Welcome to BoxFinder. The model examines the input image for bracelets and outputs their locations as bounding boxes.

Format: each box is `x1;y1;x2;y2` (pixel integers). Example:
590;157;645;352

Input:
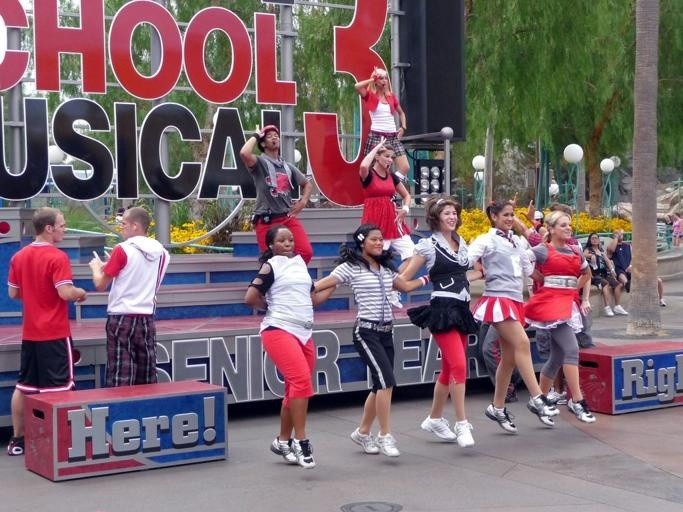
251;133;261;140
372;74;378;82
400;126;407;131
418;275;430;288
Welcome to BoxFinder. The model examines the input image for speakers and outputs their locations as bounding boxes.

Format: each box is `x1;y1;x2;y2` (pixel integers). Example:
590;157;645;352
397;0;467;144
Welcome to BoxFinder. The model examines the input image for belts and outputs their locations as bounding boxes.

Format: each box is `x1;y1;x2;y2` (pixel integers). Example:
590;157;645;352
263;310;313;331
369;130;398;138
353;318;394;333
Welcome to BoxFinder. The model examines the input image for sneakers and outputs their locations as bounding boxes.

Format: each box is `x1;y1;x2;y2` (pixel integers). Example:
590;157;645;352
614;304;628;316
605;306;614;319
392;289;403;310
485;403;520;432
453;420;475;449
530;395;561;417
350;427;379;455
568;398;596;423
525;399;555;428
288;437;312;466
658;298;666;307
374;431;399;458
270;438;298;466
548;387;567;406
420;416;458;441
503;390;518;404
6;436;25;458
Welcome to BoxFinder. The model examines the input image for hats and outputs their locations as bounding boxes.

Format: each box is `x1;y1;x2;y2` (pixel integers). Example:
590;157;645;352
260;124;279;133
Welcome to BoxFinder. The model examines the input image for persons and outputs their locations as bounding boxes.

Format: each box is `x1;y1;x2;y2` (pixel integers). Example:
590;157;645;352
665;215;673;226
509;192;597;405
672;213;683;247
524;210;597;424
534;211;545;225
240;123;313;267
88;208;171;388
358;136;415;274
7;207;87;456
478;322;521;403
468;200;561;433
399;197;483;448
315;225;431;457
244;225;344;469
607;228;667;306
355;66;410;183
583;233;630;317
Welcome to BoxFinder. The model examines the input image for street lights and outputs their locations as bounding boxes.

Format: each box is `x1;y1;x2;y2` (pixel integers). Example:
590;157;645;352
600;156;620;233
472;155;485;209
564;144;583;235
